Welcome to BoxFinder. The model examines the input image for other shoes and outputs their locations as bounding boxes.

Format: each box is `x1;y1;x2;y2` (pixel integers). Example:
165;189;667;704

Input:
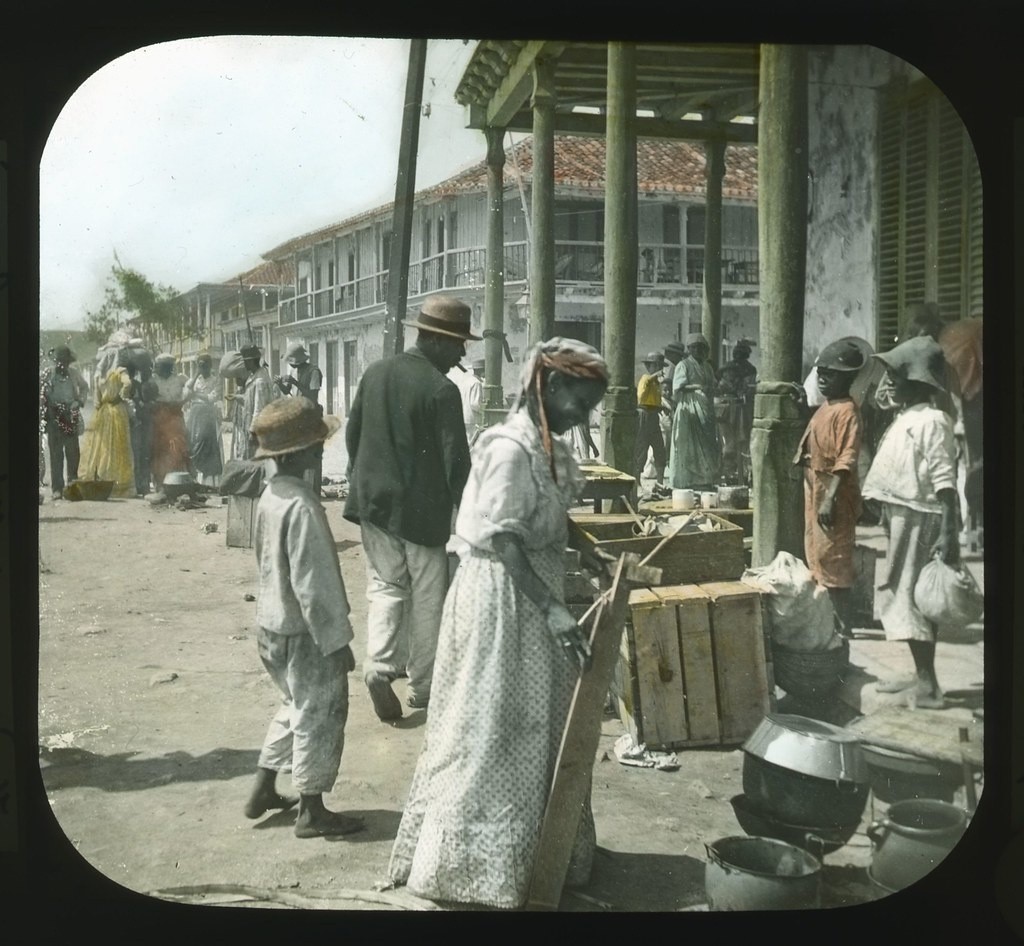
637;485;652;500
652;486;673;499
52;491;62;499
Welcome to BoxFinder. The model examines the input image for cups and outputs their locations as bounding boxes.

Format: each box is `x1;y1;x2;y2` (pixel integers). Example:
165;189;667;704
672;489;696;512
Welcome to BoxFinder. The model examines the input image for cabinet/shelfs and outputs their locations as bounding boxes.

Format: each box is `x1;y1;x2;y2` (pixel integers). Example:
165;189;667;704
605;579;771;750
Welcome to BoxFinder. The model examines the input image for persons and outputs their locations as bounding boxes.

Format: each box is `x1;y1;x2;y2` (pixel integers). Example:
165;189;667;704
632;334;760;492
42;332;322;499
244;395;367;839
342;296;482;721
860;336;984;708
391;337;618;909
790;337;866;637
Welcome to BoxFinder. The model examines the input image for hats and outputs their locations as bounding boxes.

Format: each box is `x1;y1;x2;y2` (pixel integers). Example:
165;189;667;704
240;344;261;361
662;340;687;357
642;352;669;367
154;353;175;365
400;296;483;341
869;336;947;395
252;397;341;460
687;333;706;347
810;342;863;370
48;346;76;363
281;343;309;365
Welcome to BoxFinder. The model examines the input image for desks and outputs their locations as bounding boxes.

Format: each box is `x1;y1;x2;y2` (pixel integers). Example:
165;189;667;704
576;458;639;514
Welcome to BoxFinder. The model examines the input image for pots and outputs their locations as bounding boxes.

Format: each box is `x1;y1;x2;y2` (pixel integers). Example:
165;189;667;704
863;742;982;806
704;836;822;911
69;480;115;501
740;750;870;831
162;482;199;504
731;793;863;859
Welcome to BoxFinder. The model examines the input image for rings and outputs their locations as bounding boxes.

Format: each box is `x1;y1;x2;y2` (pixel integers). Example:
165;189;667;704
564;641;570;647
